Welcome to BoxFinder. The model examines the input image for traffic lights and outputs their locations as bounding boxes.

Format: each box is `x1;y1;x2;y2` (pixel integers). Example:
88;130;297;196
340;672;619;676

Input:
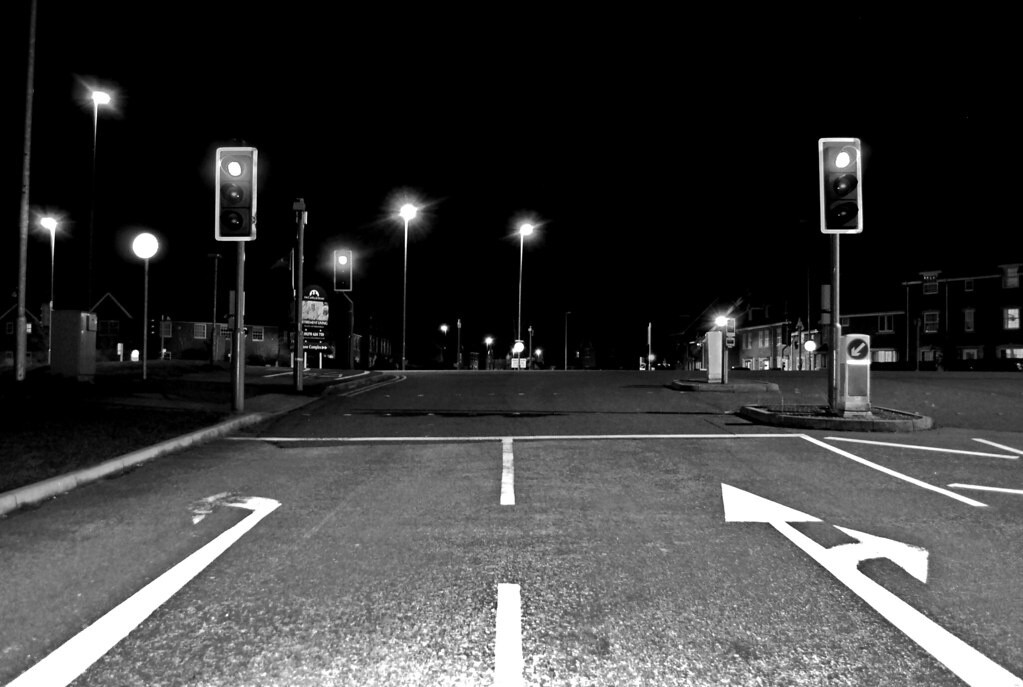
215;147;257;241
334;249;353;292
819;138;863;234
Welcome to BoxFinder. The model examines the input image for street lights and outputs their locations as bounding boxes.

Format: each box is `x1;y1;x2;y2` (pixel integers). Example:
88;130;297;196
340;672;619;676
401;204;416;370
84;90;111;313
564;311;572;370
518;224;534;371
40;217;57;366
133;232;160;378
